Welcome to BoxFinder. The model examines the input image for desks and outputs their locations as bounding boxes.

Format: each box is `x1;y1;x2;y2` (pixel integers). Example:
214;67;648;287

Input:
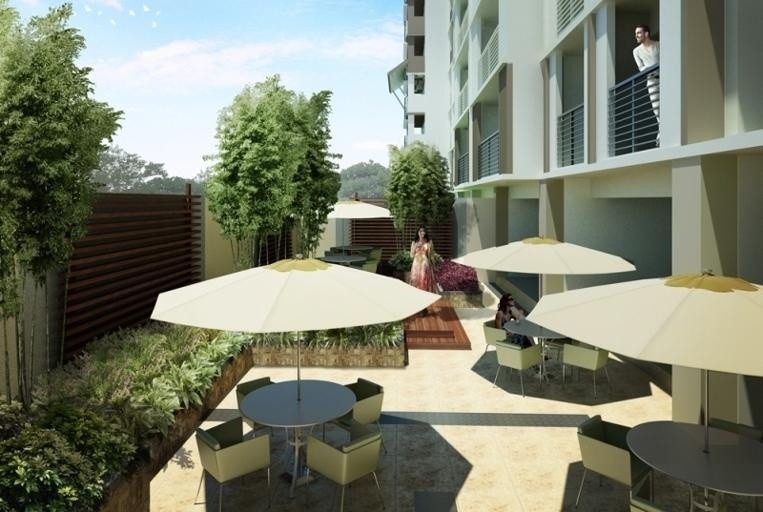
626;420;763;512
239;380;356;500
503;319;568;384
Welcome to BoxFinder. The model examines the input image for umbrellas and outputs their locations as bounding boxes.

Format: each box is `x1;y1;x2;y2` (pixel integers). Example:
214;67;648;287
148;257;442;400
524;267;763;506
450;235;635;303
316;199;396;246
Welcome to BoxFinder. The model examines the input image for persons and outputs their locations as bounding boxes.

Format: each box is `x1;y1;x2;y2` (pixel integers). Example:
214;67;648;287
631;24;660;149
495;293;541;373
409;225;435;292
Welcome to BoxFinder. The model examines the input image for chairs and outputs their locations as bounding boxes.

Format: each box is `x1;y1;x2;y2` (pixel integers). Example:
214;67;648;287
562;343;614;400
630;473;667;512
194;416;271;512
575;415;654;509
306;419;386;511
235;376;277;438
322;378;388;455
315;247;374;269
482;319;518;353
492;340;543;397
